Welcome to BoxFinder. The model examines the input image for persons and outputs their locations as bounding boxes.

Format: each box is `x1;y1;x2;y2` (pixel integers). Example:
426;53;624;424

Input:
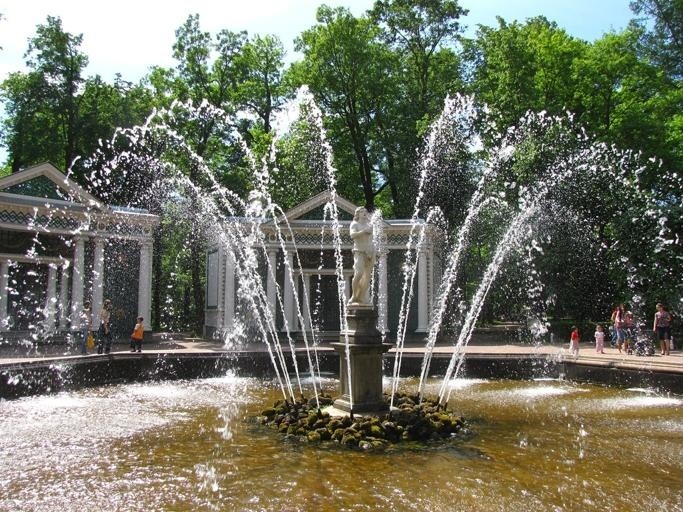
79;301;92;355
653;303;672;355
610;304;634;355
348;206;375;304
130;317;144;352
98;299;113;354
568;326;579;356
595;325;605;354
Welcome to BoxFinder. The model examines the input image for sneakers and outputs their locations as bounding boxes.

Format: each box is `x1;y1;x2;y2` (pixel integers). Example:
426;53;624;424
132;349;141;352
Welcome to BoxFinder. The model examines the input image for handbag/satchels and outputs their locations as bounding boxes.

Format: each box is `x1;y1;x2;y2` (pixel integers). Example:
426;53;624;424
131;329;141;340
88;336;94;348
